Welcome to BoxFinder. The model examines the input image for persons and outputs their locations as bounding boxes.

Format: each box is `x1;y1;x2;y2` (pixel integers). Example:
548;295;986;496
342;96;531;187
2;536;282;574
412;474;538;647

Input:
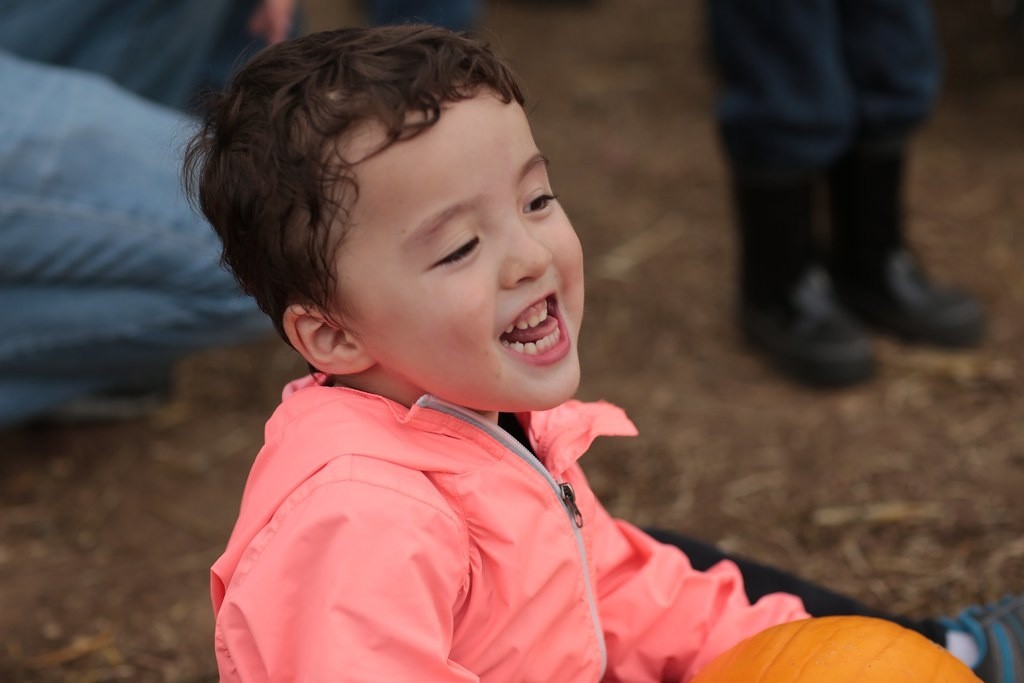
180;23;1024;683
0;0;296;433
701;0;993;387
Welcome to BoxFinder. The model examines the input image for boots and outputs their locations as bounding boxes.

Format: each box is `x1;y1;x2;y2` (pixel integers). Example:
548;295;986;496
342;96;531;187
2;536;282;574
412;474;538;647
733;158;878;382
830;145;986;342
